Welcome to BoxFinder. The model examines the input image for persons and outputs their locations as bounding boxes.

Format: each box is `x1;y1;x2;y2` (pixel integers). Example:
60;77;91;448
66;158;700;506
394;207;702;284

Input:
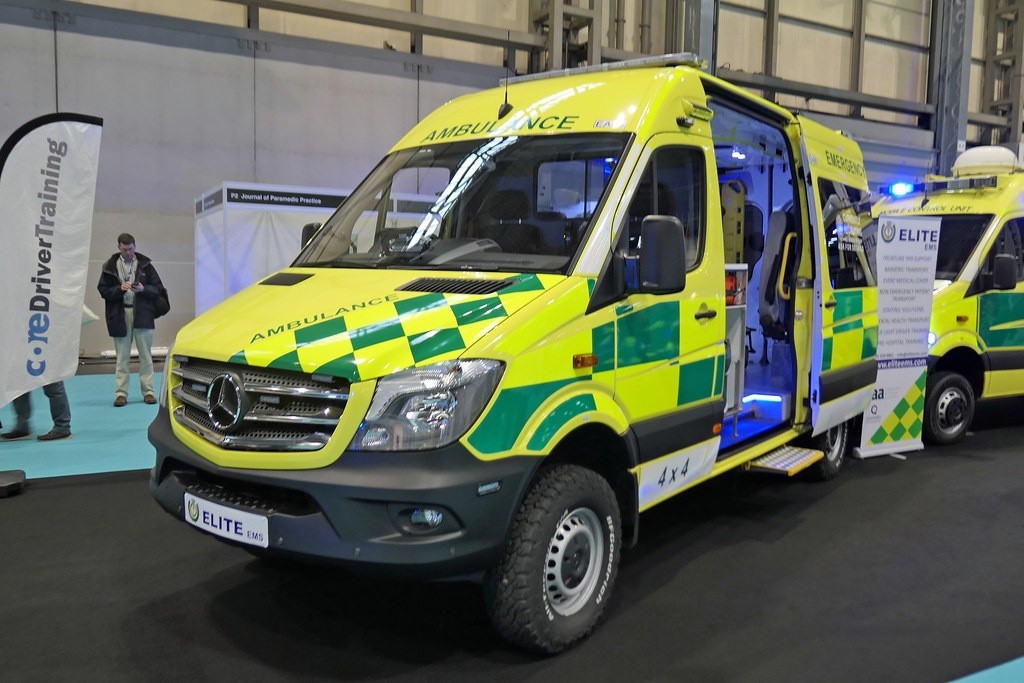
0;381;73;442
97;231;159;406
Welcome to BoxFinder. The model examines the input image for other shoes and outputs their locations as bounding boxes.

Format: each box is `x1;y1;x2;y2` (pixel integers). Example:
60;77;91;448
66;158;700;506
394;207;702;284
36;430;71;439
0;433;32;441
144;395;156;404
114;397;126;406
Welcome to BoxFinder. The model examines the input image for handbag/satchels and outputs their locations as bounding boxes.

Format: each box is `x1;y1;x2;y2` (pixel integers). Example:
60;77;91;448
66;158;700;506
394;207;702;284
153;288;170;317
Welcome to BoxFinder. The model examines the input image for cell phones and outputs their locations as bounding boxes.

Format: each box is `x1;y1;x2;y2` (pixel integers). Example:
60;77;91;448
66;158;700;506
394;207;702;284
131;281;140;289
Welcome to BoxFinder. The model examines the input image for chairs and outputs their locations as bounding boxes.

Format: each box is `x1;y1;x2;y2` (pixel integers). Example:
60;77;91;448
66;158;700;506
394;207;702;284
624;183;676;248
478;189;545;255
960;236;998;272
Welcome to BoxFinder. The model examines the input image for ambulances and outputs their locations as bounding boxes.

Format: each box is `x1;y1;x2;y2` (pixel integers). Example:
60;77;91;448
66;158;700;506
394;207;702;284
862;145;1023;448
146;49;879;659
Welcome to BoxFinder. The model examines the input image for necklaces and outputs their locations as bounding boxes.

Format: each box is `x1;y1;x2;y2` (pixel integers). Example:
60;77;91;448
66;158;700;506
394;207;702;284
119;258;135;284
